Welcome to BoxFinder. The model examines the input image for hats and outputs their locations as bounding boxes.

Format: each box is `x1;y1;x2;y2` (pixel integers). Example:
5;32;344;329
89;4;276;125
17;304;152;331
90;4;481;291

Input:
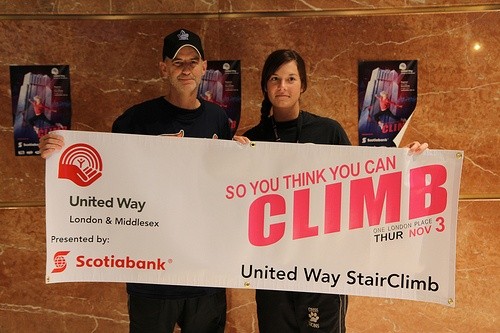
164;29;204;61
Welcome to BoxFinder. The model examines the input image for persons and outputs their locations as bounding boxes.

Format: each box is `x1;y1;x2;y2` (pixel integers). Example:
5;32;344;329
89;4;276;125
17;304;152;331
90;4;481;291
28;96;63;135
374;90;407;131
38;28;236;333
200;91;236;129
233;49;429;333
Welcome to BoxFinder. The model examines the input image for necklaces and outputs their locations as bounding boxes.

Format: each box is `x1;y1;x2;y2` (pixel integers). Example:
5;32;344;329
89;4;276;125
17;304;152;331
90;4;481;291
271;114;303;144
